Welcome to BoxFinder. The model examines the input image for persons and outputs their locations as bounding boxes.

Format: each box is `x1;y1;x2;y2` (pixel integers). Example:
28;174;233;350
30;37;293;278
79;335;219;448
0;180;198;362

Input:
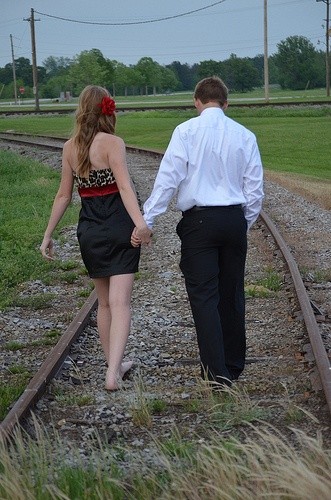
131;77;264;388
39;84;152;392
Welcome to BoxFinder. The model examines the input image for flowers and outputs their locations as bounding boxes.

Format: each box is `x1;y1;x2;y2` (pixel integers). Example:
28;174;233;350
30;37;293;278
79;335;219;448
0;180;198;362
97;96;116;116
